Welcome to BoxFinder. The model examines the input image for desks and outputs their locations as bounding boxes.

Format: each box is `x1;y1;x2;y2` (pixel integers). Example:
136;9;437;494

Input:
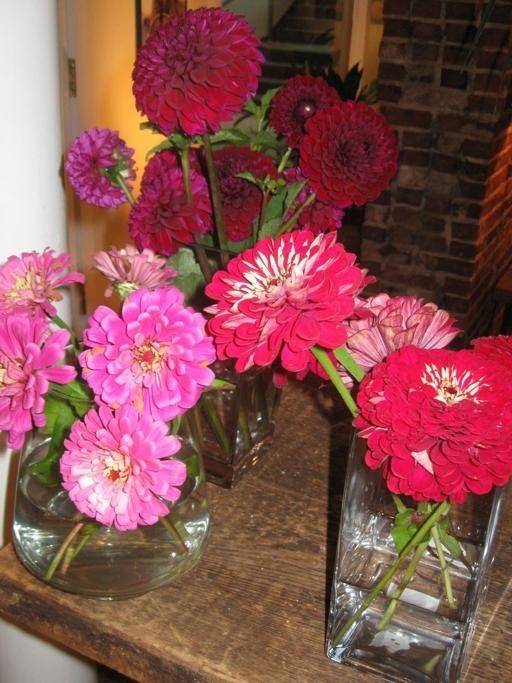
0;302;512;683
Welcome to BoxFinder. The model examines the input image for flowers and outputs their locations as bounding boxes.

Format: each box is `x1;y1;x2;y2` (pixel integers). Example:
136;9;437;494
60;6;405;463
2;247;218;583
199;226;512;650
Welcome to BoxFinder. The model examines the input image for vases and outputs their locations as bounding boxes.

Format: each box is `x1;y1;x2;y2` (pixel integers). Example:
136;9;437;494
11;389;208;602
320;431;504;682
165;341;284;485
